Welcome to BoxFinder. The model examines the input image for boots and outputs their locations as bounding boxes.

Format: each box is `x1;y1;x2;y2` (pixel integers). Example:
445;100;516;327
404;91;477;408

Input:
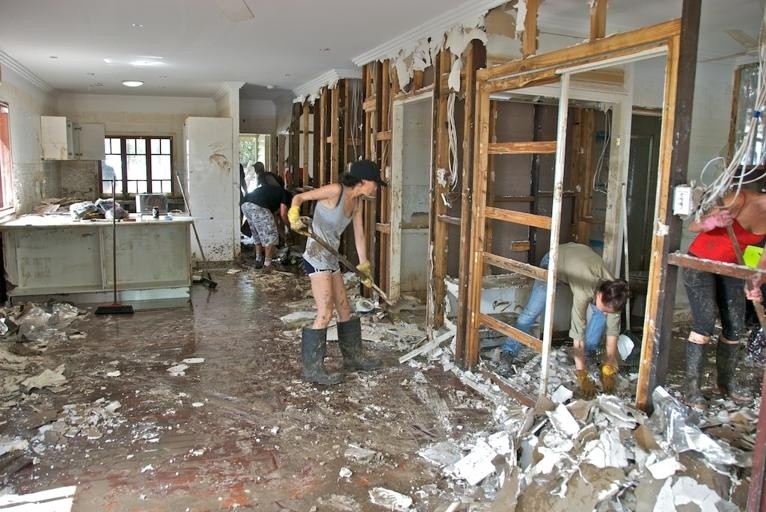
716;332;754;403
299;325;345;386
336;317;383;372
685;331;712;409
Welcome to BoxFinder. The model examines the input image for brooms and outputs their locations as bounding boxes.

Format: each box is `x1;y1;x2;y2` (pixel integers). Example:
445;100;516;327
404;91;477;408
94;176;135;314
176;174;218;288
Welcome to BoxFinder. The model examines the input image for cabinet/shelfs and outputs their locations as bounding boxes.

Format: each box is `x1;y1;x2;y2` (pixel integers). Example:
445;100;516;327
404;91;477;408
65;116;105;162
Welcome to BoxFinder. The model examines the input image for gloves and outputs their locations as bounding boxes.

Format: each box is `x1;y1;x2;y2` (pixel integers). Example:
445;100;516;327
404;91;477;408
602;363;619;394
287;206;306;231
700;209;734;233
355;261;375;288
574;369;596;399
743;280;765;303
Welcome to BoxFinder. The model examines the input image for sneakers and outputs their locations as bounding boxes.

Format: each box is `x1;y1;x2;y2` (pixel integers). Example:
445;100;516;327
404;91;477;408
255;259;264;268
499;349;515;377
262;263;279;274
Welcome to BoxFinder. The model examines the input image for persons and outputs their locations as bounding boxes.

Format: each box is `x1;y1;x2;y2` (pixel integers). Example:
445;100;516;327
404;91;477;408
679;188;765;412
494;240;634;402
285;158;389;387
251;161;286;218
239;184;303;270
238;163;247;224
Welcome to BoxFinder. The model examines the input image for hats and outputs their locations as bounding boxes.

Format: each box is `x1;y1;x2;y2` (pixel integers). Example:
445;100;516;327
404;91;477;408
349;160;388;188
